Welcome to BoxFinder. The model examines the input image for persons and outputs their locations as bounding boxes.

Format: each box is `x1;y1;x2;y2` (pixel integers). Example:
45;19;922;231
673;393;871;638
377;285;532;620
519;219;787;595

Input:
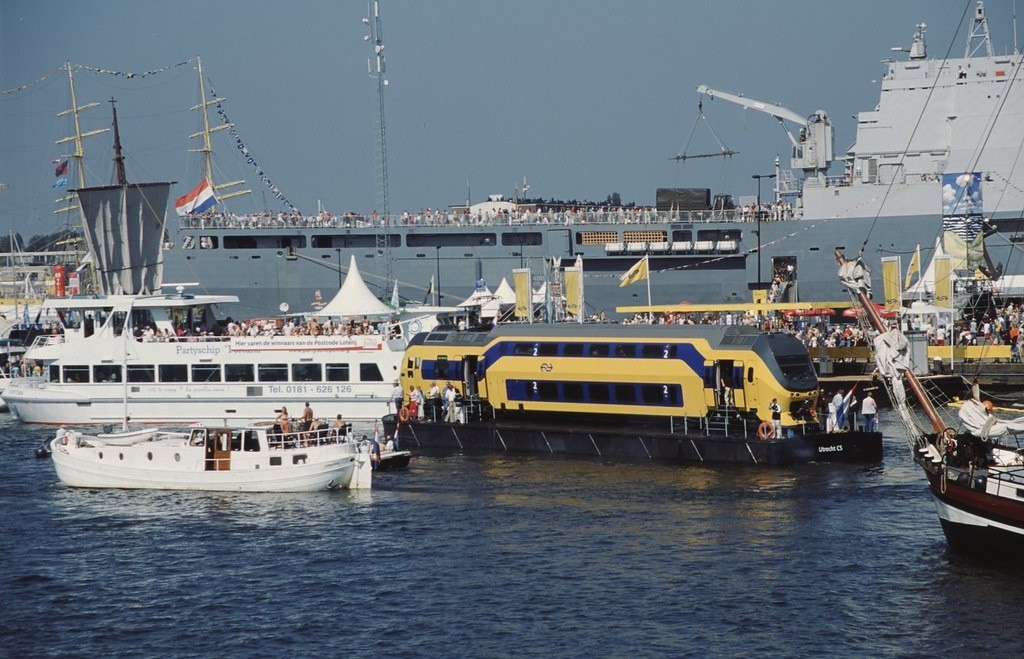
861;392;877;432
896;302;1024;363
737;200;792;223
457;319;466;332
6;356;45;378
134;316;401;343
507;311;881;350
358;435;397;456
384;378;465;425
769;399;784;439
816;389;845;432
175;203;660;232
275;402;345;446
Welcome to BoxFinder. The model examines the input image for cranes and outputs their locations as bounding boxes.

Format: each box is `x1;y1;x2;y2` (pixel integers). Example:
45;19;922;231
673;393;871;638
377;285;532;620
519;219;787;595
669;84;835;179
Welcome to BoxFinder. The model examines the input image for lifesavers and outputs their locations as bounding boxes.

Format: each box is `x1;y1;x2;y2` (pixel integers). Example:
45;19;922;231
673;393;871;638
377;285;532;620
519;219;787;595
758;422;774;438
399;407;410;422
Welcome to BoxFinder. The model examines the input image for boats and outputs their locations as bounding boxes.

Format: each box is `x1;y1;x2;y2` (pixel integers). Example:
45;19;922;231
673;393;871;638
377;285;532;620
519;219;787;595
0;0;1024;424
833;249;1024;567
373;449;411;474
381;322;883;466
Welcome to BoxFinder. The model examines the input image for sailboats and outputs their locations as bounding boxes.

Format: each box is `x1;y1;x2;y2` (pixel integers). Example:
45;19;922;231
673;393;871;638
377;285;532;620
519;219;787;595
50;328;373;491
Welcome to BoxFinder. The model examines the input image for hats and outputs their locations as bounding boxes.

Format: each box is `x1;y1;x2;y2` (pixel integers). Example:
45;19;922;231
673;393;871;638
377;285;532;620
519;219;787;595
1010;302;1014;306
971;318;978;321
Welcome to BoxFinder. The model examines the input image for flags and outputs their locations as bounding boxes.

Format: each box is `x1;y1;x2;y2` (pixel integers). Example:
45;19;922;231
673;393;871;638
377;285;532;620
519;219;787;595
373;421;382;472
934;255;953;308
836;382;859;430
564;266;584;313
175;177;221;225
51;157;68;188
880;256;903;311
618;253;649;288
905;243;921;290
393;421;400;452
512;269;532;317
24;303;30;329
